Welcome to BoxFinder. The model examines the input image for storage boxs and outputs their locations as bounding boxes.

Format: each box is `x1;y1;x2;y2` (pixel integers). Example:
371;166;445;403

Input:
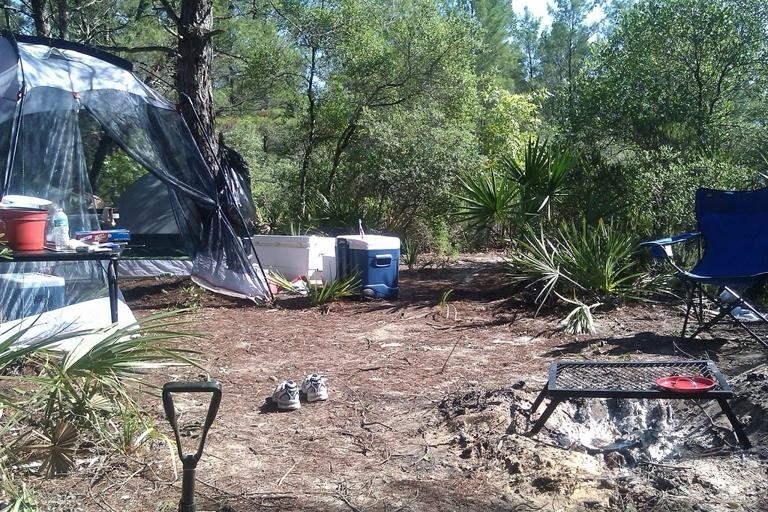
335;234;401;301
1;273;66;321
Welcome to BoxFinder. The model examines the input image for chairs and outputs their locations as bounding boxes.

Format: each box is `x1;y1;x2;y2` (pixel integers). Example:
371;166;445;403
640;188;768;349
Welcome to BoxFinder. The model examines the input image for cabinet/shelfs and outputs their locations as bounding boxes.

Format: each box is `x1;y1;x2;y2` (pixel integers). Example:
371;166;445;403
251;235;336;286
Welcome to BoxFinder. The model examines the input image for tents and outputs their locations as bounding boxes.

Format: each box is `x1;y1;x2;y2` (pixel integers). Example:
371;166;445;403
0;25;271;323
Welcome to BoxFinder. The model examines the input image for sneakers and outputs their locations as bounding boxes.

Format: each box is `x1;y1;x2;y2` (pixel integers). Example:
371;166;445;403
271;373;329;411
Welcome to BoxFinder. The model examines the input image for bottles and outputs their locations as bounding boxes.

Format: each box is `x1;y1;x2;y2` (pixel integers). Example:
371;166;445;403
52;208;69;251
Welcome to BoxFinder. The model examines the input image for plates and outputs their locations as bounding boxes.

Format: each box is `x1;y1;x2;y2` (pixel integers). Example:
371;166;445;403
656;376;717;394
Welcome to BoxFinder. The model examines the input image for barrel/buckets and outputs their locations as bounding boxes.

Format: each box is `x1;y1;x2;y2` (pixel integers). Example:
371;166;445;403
1;209;49;251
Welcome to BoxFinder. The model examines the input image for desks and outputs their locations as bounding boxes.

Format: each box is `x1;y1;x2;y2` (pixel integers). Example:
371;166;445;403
1;252;120;323
530;359;752;450
